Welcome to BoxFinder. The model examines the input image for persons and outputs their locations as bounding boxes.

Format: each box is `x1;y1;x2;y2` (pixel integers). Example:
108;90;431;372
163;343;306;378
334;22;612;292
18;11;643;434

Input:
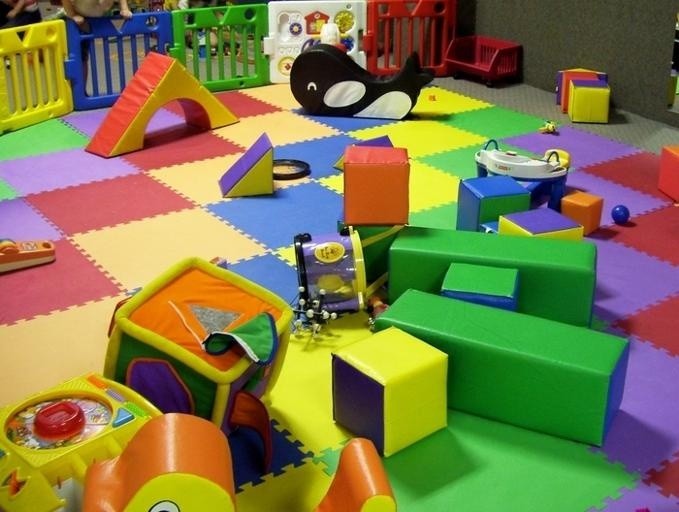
0;0;239;97
0;0;240;96
